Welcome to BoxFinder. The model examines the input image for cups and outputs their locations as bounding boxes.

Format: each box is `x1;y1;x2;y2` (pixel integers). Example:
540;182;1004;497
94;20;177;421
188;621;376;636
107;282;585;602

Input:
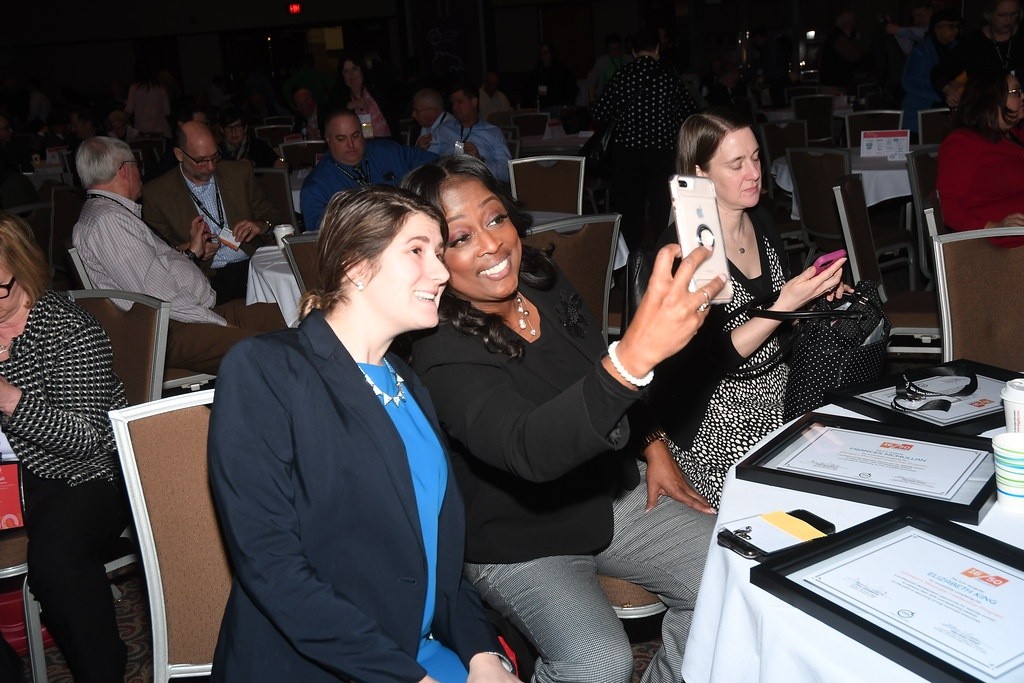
1000;378;1024;432
273;224;295;251
991;432;1024;509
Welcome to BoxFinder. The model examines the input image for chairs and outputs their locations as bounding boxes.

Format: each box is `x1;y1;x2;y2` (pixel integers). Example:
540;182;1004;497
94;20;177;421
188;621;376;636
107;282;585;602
0;68;1024;683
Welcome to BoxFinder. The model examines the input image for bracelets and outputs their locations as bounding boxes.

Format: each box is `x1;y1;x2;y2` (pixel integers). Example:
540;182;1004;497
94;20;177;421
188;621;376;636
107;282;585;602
264;218;271;226
639;429;670;454
608;341;654;386
184;248;199;264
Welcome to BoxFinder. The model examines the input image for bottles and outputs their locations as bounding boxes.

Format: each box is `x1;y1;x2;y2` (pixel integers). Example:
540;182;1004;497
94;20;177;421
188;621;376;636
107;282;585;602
301;122;309;141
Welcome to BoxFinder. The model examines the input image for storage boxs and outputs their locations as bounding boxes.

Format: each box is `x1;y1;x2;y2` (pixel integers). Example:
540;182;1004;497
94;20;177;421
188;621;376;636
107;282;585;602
0;432;25;530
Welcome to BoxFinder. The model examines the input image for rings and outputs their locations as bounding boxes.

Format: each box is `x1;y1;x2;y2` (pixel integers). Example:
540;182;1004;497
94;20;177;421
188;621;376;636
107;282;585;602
698;289;710;312
250;228;251;230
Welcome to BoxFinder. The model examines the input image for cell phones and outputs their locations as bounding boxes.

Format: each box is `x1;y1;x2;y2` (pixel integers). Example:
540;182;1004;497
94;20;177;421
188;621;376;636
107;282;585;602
668;174;734;305
717;509;836;558
810;249;847;278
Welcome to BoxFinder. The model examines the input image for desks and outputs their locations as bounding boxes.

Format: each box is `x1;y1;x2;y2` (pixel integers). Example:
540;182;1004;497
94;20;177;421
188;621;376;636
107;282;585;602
260;169;311;214
245;211;630;329
518;131;592;147
772;144;940;233
681;373;1024;683
764;106;852;125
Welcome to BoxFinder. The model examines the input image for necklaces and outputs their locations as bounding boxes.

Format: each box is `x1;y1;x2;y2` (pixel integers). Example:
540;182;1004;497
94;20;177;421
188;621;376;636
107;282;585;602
988;28;1013;69
738;247;745;254
358;358;406;407
513;293;536;341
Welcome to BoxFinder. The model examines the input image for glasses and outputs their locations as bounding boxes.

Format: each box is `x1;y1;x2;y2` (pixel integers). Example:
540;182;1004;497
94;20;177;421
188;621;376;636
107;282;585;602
0;276;17;299
119;159;144;171
223;124;244;132
180;146;223;167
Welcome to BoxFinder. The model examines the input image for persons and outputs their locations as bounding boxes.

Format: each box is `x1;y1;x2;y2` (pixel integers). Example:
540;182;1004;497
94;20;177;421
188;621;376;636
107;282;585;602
138;121;281;305
210;183;525;683
0;54;513;204
0;210;133;683
529;24;789;133
589;28;699;288
299;108;440;230
72;136;289;376
397;152;728;683
647;112;854;515
816;0;1024;248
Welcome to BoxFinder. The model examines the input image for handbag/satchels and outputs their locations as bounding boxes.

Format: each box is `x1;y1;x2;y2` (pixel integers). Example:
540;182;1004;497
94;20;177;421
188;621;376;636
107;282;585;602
577;122;616;170
718;283;892;424
